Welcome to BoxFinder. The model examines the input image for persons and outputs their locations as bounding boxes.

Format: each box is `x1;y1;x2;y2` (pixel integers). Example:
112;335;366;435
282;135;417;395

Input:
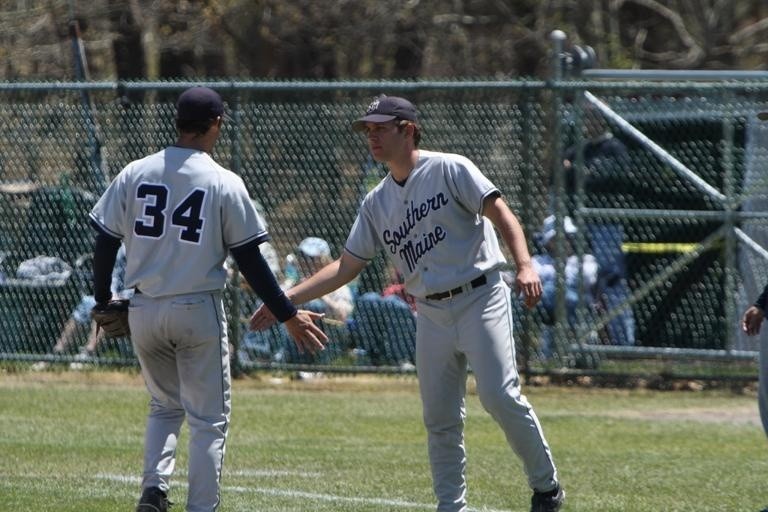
221;197;282;369
524;212;602;389
86;84;331;512
353;260;421;374
246;234;356;385
561;95;638;360
28;242;133;373
246;93;568;512
738;280;766;443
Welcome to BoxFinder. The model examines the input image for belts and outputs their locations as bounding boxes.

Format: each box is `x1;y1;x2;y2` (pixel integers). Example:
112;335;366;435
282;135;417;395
425;273;486;300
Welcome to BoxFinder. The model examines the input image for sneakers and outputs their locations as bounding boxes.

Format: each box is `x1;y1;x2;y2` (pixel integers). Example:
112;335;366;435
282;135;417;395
267;371;327;383
136;487;169;511
531;484;566;512
29;353;98;373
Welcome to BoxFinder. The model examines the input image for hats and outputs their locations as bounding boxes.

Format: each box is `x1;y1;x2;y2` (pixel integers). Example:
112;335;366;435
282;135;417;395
352;96;416;132
298;237;331;257
174;86;237;127
542;215;578;245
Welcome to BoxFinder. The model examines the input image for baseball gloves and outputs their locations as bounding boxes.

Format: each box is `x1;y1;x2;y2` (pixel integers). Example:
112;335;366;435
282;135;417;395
92;301;129;338
